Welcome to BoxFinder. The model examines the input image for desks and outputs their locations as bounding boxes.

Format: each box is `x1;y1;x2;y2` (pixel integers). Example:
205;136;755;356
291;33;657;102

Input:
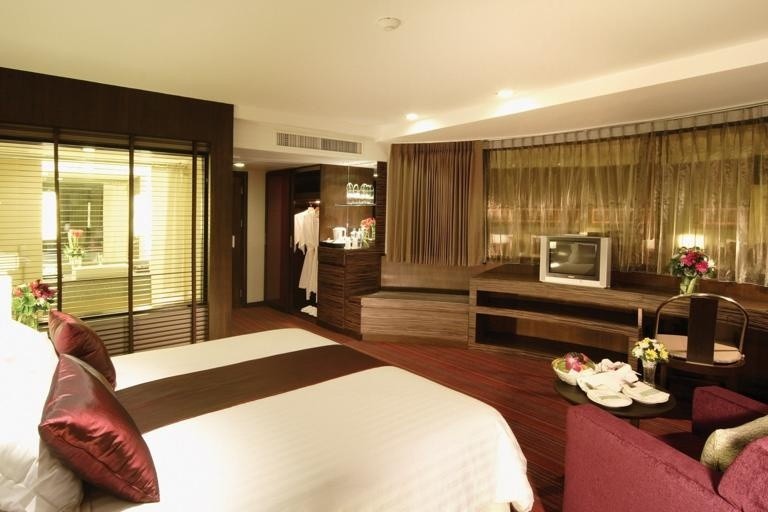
552;369;678;431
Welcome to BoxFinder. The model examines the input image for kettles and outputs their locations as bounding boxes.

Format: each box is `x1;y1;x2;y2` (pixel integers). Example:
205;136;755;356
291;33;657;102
332;227;346;242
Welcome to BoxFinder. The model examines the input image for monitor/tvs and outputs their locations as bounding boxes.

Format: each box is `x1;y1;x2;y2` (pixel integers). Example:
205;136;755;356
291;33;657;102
538;234;612;289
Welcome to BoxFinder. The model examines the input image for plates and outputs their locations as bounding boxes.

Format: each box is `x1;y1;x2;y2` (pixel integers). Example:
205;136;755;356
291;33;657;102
575;368;670;409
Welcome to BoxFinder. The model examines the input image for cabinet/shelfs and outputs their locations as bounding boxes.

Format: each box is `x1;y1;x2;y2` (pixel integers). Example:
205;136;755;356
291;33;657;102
317;242;382;341
263;163;372;325
467;260;768;381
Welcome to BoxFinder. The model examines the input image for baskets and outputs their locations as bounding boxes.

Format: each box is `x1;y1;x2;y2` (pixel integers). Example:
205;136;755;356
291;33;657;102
552;353;599;386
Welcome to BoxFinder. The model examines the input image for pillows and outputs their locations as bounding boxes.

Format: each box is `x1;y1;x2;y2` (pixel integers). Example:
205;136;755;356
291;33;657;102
46;308;121;392
39;349;163;502
699;410;768;470
0;321;86;511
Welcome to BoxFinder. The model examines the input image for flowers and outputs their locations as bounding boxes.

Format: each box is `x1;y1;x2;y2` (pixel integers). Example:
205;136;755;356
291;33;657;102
11;279;54;328
630;335;669;361
668;240;716;279
68;229;84;257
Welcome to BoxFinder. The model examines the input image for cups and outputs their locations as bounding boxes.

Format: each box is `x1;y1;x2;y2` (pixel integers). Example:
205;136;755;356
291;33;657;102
343;238;363;249
345;189;374;204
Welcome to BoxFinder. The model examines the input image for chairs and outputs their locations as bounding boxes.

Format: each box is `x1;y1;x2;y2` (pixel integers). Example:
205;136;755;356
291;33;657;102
651;292;754;387
559;382;768;511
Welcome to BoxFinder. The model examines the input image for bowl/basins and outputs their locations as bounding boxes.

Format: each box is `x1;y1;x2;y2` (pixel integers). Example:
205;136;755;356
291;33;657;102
550;357;594;385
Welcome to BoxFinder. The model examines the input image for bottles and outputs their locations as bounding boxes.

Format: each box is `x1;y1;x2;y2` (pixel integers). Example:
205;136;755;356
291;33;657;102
350;227;369;242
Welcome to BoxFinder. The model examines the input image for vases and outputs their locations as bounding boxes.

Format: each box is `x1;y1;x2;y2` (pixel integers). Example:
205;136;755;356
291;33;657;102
70;254;83;270
677;272;705;296
642;359;659;384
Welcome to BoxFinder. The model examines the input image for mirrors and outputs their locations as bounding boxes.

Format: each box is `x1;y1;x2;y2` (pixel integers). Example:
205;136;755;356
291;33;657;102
41;181;105;254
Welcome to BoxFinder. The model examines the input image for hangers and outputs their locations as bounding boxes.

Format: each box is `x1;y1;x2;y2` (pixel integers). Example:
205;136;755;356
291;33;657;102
295;203;322;216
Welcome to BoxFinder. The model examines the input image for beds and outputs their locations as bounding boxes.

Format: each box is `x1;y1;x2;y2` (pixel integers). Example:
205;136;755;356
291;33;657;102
0;317;544;512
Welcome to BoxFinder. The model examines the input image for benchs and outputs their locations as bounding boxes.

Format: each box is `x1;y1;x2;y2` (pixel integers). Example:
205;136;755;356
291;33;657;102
361;254;480;343
